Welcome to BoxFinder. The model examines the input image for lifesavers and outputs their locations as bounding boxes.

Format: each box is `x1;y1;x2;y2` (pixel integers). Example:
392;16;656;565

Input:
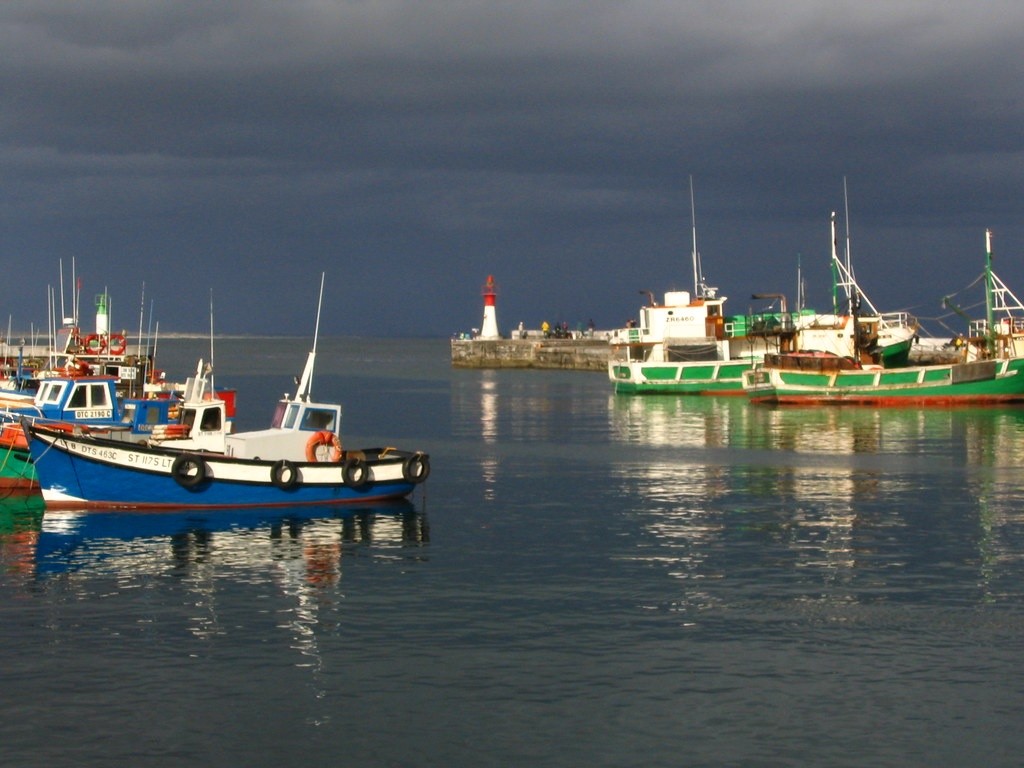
171;453;206;489
304;430;343;463
148;423;191;440
84;333;106;355
55;367;84;378
270;458;298;490
108;332;126;355
402;453;431;484
341;458;368;487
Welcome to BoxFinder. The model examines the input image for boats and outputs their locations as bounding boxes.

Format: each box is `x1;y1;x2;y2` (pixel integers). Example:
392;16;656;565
777;176;920;368
763;227;1024;403
608;178;816;395
19;271;431;509
0;256;237;487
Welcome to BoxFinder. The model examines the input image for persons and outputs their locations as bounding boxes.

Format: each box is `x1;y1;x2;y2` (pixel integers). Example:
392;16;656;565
576;320;584;338
627;319;637;328
542;322;549;335
955;333;964;351
79;361;93;376
519;321;524;340
588;319;594;337
563;322;572;339
554;321;561;336
